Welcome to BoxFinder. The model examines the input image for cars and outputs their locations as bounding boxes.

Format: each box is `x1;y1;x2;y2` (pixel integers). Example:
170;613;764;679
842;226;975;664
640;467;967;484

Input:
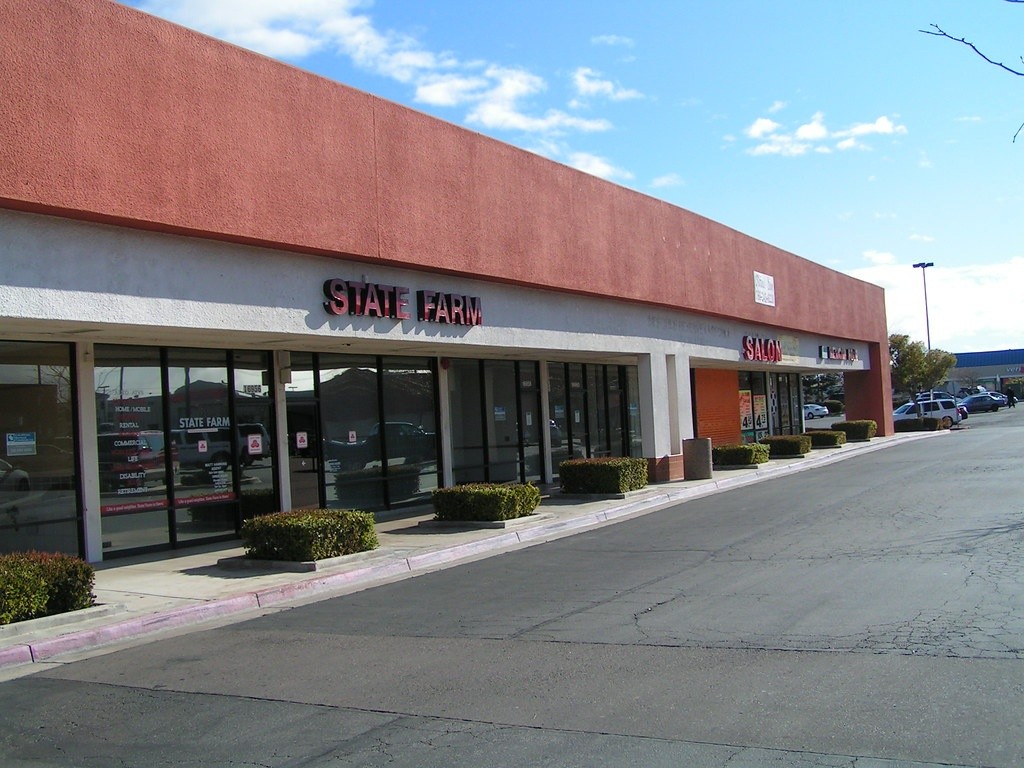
910;392;959;403
367;422;439;460
16;423;271;490
893;399;961;430
977;391;1017;405
957;386;987;401
0;458;31;500
804;404;828;420
961;391;1006;413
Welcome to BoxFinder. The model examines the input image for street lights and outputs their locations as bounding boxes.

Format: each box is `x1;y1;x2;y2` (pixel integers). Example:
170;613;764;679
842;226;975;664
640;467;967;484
913;262;934;397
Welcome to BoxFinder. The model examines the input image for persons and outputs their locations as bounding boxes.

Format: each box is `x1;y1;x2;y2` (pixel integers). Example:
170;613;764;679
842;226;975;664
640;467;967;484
1006;387;1015;408
